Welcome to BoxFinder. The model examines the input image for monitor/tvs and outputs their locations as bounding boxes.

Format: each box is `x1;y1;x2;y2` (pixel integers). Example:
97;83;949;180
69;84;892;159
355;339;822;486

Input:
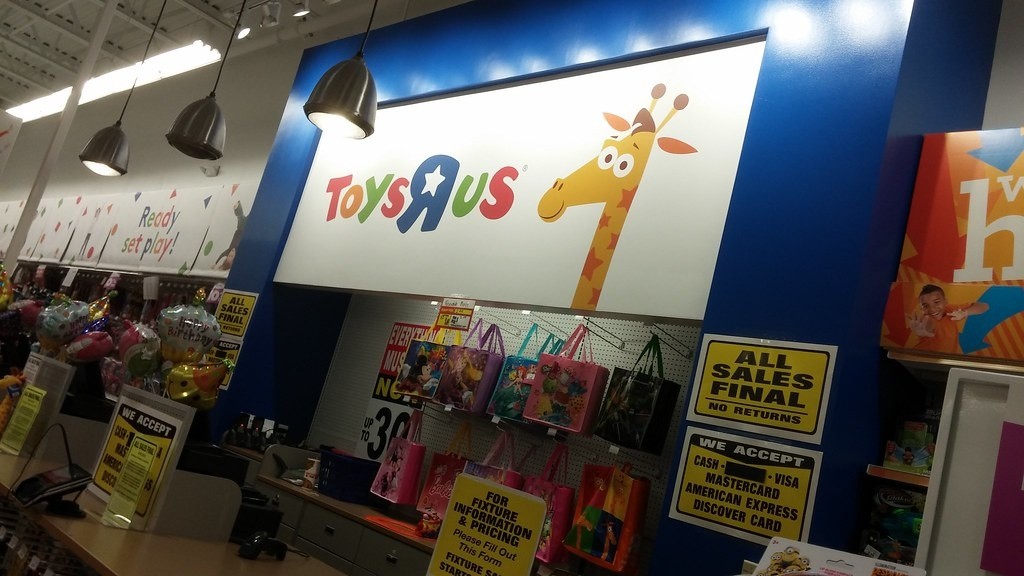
13;463;94;507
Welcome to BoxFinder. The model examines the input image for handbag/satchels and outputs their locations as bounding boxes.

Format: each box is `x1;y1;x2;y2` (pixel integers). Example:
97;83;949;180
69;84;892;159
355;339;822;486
521;323;610;439
591;333;681;458
370;408;426;507
561;457;652;576
392;314;571;438
415;420;577;565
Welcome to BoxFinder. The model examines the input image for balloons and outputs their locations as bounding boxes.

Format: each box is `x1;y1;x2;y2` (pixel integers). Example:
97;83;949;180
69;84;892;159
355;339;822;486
0;262;235;411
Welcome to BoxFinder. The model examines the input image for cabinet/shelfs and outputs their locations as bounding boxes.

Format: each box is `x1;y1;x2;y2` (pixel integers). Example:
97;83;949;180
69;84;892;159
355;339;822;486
864;349;1024;489
223;443;434;576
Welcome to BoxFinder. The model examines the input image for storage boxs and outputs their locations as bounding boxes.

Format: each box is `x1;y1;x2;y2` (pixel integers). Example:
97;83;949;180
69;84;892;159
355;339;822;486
879;130;1024;365
312;448;382;508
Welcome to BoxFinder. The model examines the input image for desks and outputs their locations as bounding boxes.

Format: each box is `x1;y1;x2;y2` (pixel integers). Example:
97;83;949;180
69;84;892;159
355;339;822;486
0;445;347;576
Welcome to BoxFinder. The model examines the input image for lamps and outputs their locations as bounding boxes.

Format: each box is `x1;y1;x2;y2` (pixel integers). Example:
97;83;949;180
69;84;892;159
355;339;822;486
77;0;379;179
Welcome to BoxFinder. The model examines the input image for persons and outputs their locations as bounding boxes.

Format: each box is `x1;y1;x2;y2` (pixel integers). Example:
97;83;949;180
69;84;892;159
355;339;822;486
904;283;989;353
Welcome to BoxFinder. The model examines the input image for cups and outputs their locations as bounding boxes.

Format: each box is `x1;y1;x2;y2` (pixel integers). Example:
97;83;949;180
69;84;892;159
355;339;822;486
303;458;321;491
